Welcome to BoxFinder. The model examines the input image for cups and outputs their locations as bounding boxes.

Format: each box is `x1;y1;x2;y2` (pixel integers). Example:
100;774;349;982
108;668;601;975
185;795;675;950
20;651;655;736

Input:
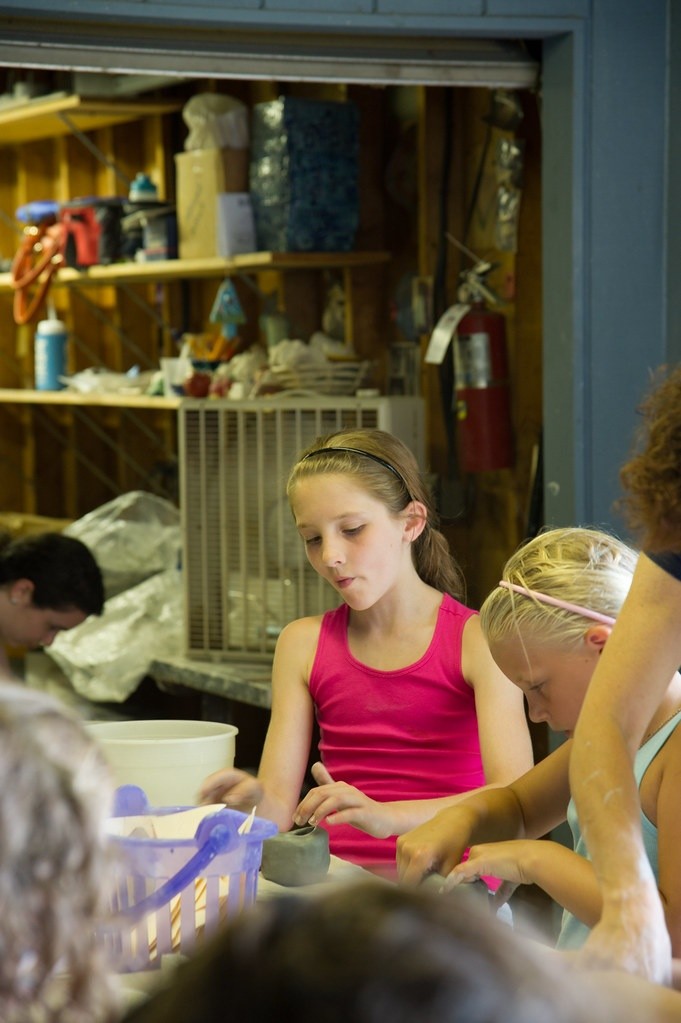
33;319;68;390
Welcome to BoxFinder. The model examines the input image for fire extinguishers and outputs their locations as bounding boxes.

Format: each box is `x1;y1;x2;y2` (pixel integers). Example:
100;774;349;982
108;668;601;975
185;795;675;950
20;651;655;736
420;258;520;470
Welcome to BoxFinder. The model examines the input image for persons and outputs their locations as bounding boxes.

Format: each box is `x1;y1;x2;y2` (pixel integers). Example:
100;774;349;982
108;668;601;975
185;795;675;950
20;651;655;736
397;524;679;980
572;347;681;983
2;687;117;1021
122;885;583;1021
2;532;106;682
198;425;541;934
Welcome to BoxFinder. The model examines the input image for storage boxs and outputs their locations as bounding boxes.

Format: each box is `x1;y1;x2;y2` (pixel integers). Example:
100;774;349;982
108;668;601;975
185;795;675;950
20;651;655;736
77;720;238;811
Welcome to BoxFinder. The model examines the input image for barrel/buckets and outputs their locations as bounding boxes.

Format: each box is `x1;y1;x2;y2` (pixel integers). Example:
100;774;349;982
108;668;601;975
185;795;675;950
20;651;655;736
82;720;238;809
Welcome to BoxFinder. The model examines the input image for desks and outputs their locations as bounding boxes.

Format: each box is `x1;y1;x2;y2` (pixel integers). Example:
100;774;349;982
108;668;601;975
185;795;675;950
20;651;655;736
256;852;390;902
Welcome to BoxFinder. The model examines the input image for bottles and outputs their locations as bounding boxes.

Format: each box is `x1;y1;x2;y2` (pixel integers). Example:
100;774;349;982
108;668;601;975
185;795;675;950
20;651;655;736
126;171;157;203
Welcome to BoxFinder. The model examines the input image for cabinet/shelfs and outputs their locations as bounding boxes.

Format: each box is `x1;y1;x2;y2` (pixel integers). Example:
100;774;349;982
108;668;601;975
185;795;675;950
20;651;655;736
1;94;420;530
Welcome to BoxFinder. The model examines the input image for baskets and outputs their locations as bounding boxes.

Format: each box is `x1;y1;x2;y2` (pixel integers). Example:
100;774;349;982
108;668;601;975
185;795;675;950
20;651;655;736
90;786;279;974
